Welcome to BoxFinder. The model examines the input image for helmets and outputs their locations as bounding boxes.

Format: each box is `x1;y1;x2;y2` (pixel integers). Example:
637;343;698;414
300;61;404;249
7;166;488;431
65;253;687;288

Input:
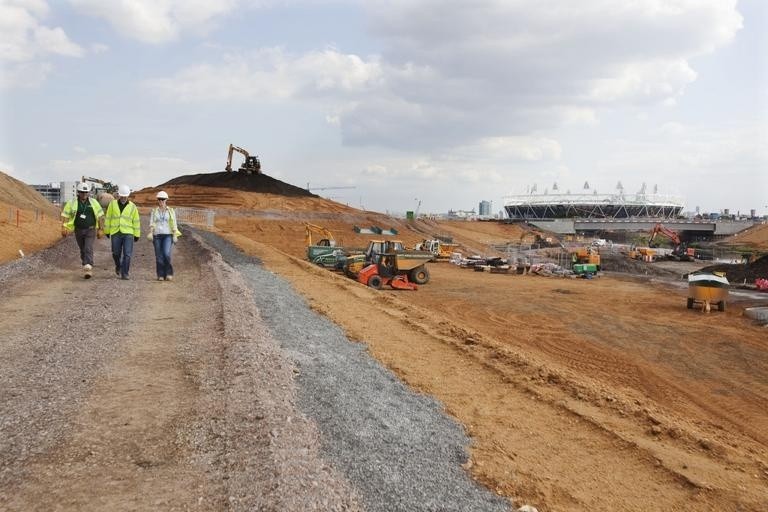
77;183;90;192
156;191;169;198
118;184;130;196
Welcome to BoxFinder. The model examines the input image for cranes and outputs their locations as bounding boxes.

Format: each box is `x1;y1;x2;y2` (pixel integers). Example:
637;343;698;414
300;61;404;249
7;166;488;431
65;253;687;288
304;182;357;194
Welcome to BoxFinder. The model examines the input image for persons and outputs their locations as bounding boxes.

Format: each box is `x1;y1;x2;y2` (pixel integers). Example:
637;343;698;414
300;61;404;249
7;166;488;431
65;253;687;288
104;184;141;280
147;191;183;280
60;182;105;279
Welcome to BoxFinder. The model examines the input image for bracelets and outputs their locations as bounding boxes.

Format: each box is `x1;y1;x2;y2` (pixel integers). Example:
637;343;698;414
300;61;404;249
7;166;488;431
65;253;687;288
99;227;103;230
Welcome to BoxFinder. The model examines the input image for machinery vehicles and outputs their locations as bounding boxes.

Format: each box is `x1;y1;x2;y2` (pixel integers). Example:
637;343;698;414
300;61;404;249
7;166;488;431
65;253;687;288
302;219;462;290
81;176;119;208
520;227;600;278
225;144;262;176
626;224;716;262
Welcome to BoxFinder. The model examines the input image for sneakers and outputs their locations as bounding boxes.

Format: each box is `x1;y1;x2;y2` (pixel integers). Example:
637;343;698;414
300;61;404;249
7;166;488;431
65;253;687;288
159;276;172;280
84;272;92;279
116;271;129;280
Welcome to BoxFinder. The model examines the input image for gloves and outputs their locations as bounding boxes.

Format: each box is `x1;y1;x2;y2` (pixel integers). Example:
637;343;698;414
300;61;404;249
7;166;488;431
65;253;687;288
134;237;139;242
173;237;178;246
97;230;104;239
147;233;153;241
106;233;110;238
62;227;68;237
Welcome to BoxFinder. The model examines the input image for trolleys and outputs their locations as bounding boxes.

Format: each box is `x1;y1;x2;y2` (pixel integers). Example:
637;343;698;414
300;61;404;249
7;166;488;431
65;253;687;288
686;271;730;312
